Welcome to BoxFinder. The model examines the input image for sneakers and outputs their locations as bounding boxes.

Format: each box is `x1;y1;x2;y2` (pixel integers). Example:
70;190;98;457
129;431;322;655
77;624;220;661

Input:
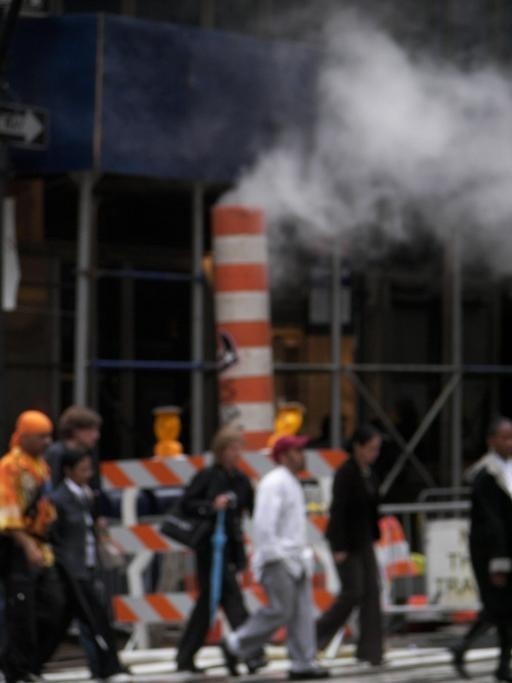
221;641;239;676
452;645;472;677
289;669;329;680
177;662;204;673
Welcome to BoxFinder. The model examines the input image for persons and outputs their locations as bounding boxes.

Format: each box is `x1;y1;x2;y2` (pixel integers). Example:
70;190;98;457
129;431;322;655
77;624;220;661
0;407;135;683
450;416;512;683
315;425;387;666
176;429;269;673
218;435;331;680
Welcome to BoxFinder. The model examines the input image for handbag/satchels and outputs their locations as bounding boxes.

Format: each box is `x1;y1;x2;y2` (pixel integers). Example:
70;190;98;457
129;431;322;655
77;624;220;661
161;511;214;547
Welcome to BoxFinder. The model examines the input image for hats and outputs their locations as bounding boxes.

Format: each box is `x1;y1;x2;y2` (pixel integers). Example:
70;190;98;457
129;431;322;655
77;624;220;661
272;435;311;461
